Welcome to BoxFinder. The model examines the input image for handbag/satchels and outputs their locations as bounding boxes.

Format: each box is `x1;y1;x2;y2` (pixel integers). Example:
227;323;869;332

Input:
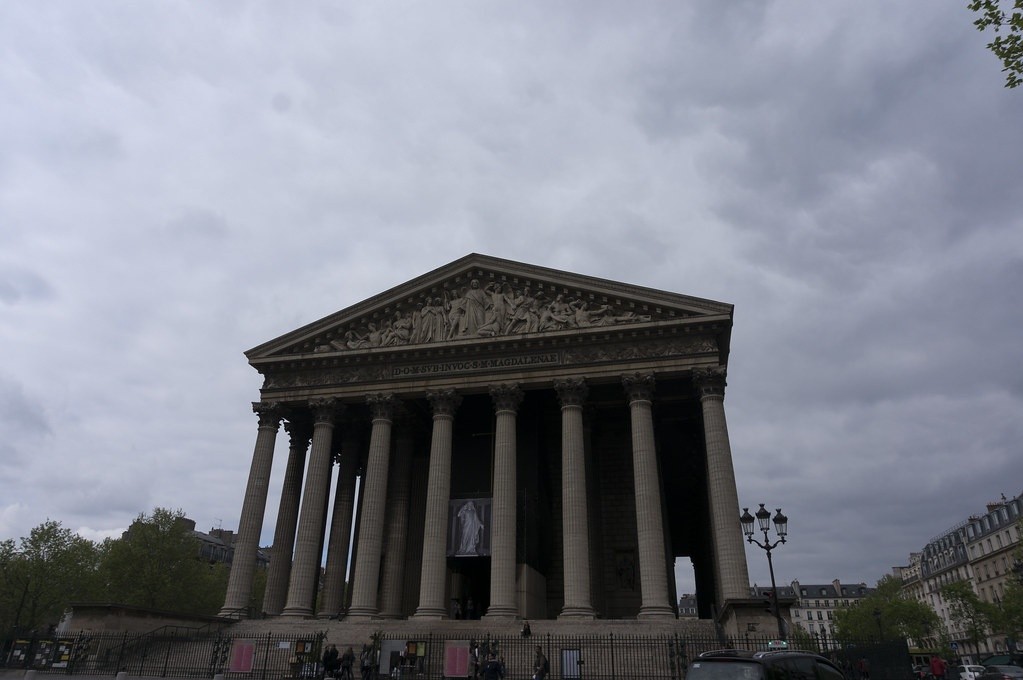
521;630;524;635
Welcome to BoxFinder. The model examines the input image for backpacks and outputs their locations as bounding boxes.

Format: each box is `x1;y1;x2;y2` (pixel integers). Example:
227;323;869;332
538;655;549;673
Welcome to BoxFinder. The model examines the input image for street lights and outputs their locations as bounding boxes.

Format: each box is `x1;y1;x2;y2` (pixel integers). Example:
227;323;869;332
738;504;791;639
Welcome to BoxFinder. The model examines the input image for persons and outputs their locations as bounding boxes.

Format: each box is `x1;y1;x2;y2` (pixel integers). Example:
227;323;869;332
361;641;426;680
523;621;531;638
532;645;548;680
477;653;503;680
322;644;355;680
930;654;947;680
456;502;485;553
345;279;638;349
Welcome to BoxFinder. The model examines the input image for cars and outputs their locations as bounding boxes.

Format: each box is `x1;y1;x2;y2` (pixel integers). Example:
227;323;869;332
687;652;846;679
975;665;1022;680
957;665;985;680
